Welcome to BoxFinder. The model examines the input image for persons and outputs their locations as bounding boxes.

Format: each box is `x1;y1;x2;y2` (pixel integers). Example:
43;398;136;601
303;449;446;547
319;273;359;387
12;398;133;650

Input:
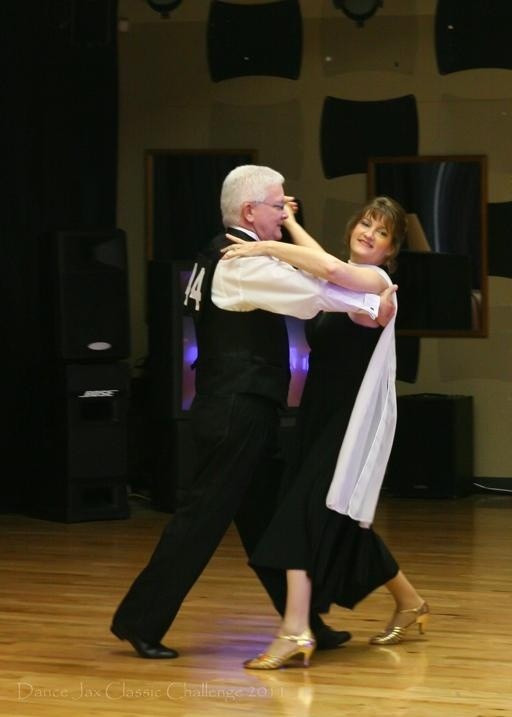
220;196;430;670
110;163;400;660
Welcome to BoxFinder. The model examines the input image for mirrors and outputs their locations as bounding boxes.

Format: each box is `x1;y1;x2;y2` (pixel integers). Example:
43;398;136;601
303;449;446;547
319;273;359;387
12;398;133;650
367;154;489;338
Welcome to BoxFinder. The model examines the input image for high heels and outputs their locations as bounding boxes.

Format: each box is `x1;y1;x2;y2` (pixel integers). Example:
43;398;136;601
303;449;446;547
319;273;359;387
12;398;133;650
372;599;430;647
245;628;316;669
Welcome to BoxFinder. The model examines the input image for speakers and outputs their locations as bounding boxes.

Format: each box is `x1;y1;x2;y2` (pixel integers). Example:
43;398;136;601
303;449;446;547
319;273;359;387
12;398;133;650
397;395;472;499
44;225;132;361
28;362;128;522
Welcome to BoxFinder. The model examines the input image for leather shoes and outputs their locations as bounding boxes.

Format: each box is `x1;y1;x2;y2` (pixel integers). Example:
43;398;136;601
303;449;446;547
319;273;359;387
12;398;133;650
110;624;178;657
313;627;351;648
